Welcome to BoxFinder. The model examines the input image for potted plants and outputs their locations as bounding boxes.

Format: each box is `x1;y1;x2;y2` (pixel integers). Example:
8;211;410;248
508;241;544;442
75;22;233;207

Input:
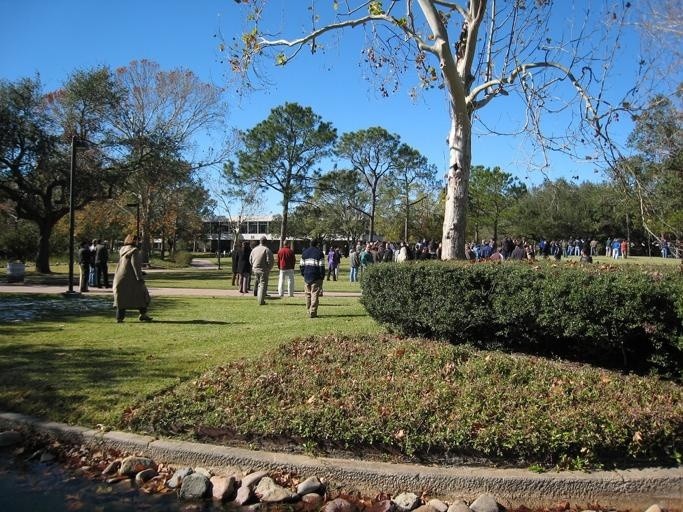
0;222;39;283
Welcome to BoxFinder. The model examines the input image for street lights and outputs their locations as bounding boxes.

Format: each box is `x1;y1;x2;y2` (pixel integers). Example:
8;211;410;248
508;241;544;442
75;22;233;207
126;203;139;239
63;136;90;297
215;221;223;270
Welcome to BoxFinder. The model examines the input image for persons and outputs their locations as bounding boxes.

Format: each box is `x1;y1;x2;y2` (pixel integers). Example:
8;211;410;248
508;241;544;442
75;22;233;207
248;236;274;306
661;239;667;258
230;245;239;286
95;241;111;289
237;241;250;294
89;239;97;287
299;238;326;319
465;238;627;262
112;234;152;323
356;237;443;280
276;239;297;297
78;241;91;293
326;243;349;283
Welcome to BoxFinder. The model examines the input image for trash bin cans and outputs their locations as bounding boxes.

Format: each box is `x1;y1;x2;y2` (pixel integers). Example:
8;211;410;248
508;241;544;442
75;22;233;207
8;257;25;283
